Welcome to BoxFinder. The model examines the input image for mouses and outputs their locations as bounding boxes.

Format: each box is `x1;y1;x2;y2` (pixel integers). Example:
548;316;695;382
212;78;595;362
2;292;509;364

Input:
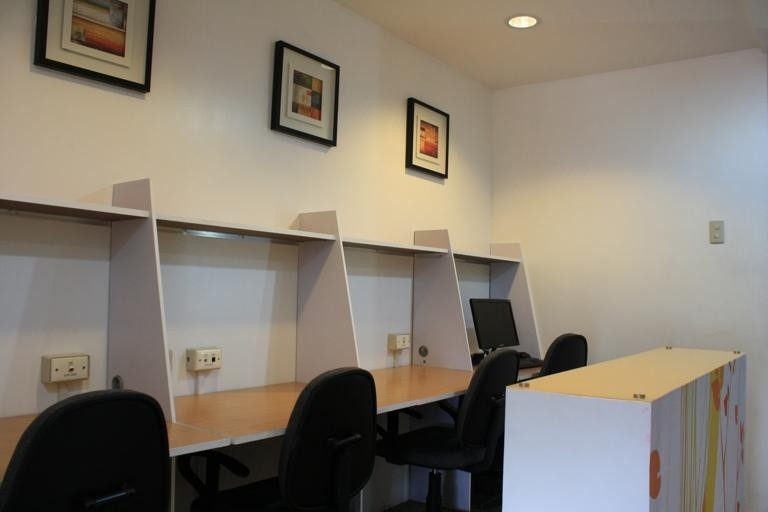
519;352;530;358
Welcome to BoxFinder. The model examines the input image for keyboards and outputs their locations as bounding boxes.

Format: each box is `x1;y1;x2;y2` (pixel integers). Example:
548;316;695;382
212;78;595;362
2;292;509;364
518;358;544;369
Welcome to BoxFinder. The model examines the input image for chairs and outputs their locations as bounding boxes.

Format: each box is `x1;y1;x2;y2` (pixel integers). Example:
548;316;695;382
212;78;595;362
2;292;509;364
2;332;590;512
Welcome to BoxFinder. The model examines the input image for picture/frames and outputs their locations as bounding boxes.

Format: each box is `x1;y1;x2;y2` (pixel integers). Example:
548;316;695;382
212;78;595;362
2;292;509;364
31;0;157;95
404;96;451;180
266;35;342;149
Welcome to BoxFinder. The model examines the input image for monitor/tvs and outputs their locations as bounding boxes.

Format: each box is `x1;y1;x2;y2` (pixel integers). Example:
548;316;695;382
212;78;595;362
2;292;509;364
469;298;520;355
